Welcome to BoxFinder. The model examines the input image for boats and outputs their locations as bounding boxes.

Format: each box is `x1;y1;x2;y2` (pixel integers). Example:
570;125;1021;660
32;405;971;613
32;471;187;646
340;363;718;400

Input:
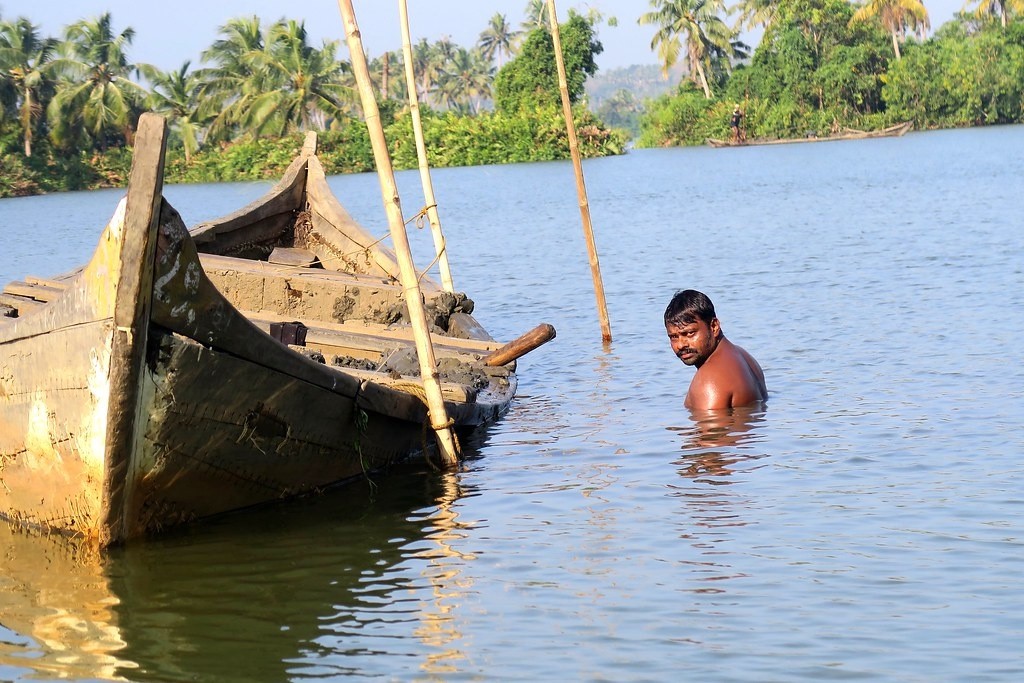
0;110;519;553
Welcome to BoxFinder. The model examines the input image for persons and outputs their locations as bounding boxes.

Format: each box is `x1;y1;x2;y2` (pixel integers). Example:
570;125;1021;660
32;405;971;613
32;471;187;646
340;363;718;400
730;104;743;143
664;290;767;410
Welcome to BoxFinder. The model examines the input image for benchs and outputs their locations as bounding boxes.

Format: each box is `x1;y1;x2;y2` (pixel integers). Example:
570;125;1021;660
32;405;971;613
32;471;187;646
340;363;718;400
198;251;410;324
0;276;516;370
